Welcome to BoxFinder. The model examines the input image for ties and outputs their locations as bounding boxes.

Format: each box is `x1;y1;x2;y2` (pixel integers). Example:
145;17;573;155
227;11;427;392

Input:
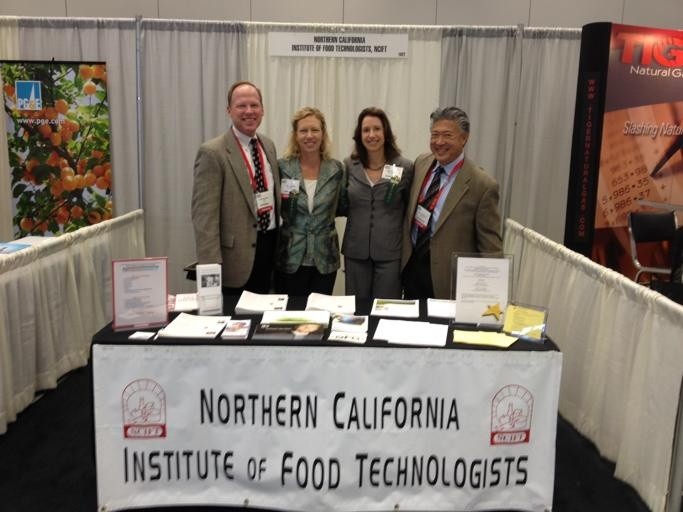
415;167;446;258
250;138;271;232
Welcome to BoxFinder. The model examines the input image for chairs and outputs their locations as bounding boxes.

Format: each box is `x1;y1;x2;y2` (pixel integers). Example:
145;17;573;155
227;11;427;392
627;209;681;288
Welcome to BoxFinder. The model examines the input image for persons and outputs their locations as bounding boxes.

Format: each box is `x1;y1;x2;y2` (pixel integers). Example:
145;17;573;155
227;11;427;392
344;107;414;315
277;106;348;296
399;107;505;299
191;81;282;295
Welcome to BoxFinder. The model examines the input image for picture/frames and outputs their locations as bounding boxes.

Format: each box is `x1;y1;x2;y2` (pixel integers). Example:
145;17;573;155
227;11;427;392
109;256;169;333
449;251;516;332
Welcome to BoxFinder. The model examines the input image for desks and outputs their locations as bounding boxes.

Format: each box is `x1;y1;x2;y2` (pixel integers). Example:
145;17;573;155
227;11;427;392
88;313;559;512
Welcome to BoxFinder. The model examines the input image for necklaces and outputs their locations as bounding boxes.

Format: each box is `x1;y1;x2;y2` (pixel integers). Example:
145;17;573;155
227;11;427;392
365;158;388;170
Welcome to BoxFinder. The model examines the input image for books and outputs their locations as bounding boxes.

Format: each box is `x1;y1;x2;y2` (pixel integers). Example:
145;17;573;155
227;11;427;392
158;290;456;347
